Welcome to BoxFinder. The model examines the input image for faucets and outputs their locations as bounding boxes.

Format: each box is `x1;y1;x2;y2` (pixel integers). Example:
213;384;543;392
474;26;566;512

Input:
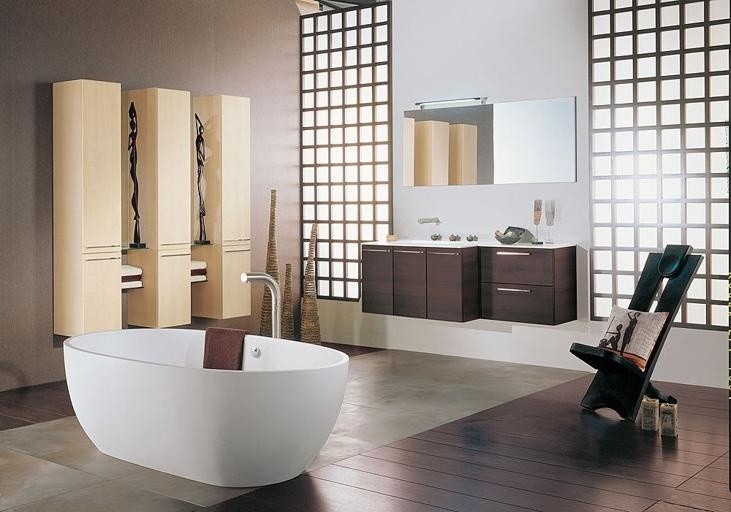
240;272;283;337
417;216;439;224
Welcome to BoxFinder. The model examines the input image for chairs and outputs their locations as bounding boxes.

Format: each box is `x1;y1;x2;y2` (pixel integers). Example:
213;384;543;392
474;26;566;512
569;244;705;423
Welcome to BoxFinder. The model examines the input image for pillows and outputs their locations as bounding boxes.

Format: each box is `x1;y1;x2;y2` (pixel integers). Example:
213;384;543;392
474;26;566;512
597;304;671;373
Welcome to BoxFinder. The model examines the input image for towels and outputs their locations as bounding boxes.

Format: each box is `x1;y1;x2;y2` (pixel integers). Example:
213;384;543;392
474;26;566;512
202;326;250;371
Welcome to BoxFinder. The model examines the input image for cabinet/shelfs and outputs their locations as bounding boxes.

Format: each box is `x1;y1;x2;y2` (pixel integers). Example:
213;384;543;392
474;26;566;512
51;78;251;341
479;246;577;325
362;245;478;323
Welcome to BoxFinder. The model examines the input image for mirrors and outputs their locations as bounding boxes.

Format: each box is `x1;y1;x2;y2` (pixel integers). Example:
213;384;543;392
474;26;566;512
403;96;577;186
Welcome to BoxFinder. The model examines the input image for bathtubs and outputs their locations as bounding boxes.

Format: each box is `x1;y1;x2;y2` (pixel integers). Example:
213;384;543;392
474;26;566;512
62;328;351;488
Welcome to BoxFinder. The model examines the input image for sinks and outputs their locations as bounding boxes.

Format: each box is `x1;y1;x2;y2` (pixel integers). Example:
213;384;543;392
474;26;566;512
390;238;461;244
